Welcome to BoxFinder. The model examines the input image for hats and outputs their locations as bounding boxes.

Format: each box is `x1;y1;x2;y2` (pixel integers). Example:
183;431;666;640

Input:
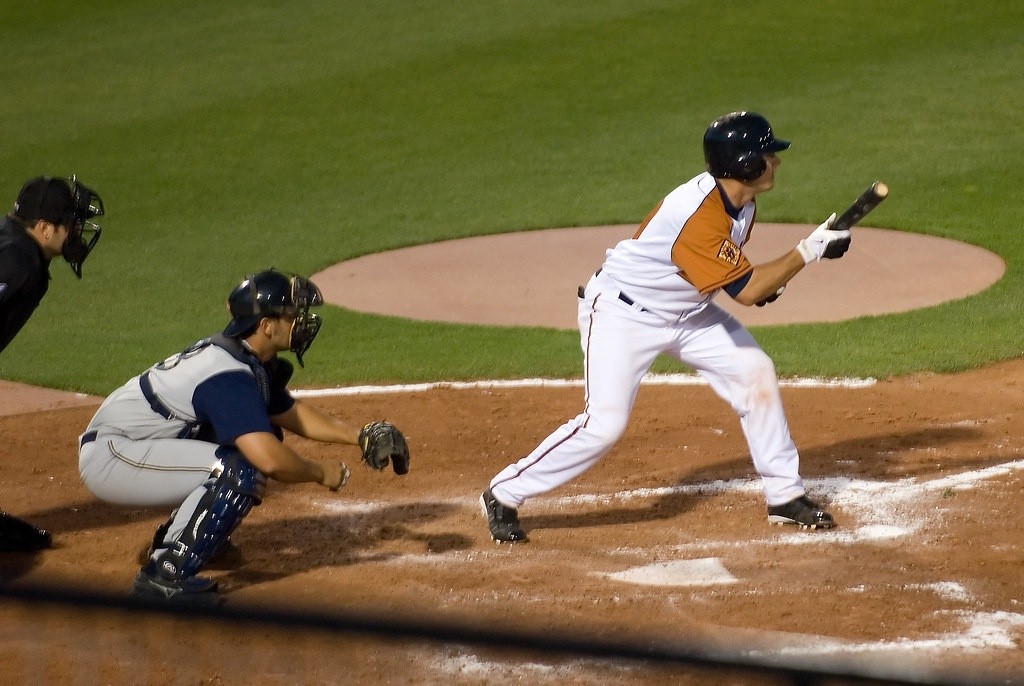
13;177;74;223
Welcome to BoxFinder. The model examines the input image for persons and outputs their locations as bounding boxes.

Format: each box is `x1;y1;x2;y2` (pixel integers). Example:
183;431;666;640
78;266;410;602
479;112;851;545
0;175;107;551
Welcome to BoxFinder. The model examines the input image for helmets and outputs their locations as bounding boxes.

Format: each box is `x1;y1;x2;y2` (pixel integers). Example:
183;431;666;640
703;111;791;181
223;271;294;337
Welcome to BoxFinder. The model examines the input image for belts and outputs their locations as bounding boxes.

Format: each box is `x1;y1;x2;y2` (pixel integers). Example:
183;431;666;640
595;268;649;312
80;431;97;450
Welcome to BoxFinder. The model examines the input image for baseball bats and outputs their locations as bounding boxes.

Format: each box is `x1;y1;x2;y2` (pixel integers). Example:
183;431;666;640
755;180;891;308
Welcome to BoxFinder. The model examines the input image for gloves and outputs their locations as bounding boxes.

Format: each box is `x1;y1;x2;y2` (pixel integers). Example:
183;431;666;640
800;212;851;260
756;283;787;306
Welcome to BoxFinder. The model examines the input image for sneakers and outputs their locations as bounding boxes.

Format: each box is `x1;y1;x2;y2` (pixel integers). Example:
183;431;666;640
0;512;51;552
478;487;527;543
134;564;218;601
768;495;835;529
149;527;239;566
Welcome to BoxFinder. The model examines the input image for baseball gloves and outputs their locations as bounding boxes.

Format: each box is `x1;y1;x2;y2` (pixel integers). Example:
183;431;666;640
358;420;411;477
